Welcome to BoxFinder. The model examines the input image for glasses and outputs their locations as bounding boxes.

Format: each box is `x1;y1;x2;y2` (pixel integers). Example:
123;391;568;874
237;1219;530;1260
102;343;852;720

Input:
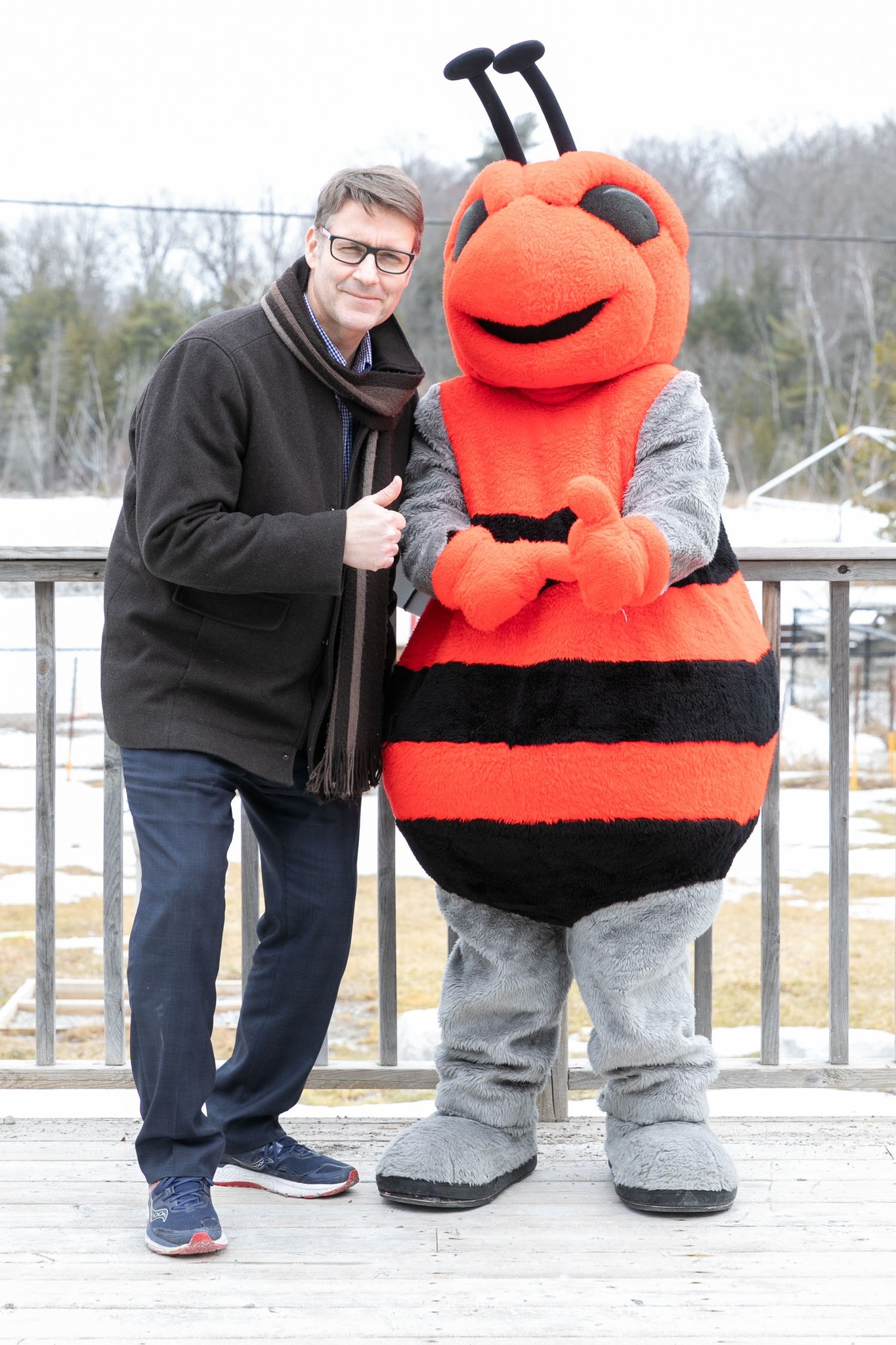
318;227;418;275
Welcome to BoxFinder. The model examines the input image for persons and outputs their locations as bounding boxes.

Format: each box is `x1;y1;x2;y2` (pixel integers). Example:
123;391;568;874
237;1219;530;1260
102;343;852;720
102;167;425;1256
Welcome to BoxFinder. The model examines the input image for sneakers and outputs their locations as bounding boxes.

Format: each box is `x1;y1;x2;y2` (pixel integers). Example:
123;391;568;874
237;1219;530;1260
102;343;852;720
144;1177;226;1257
212;1134;358;1200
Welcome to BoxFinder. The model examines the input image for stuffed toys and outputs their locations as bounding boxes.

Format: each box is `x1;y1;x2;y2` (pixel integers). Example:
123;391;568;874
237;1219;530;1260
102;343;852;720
378;41;783;1210
